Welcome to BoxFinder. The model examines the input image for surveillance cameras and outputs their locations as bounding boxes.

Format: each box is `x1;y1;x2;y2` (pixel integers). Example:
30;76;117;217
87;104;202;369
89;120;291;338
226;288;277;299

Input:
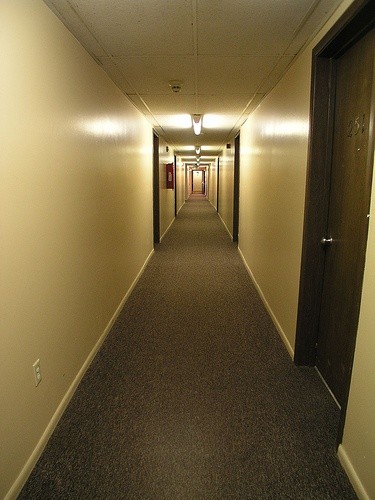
170;85;182;94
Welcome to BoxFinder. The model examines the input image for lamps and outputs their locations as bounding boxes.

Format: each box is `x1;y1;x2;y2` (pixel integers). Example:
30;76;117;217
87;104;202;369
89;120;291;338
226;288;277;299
196;146;201;156
192;115;203;137
196;157;200;163
197;164;200;167
